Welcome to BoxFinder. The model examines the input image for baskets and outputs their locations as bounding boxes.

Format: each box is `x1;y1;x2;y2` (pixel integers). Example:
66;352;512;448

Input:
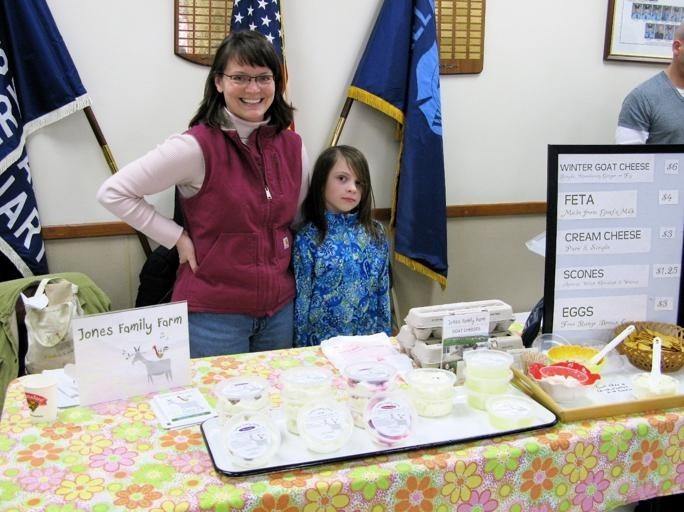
615;320;684;374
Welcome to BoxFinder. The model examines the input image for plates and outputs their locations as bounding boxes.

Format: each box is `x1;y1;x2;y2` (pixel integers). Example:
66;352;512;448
527;360;601;385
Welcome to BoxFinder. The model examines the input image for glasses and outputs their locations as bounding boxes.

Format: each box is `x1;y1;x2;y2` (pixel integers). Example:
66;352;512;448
221;73;273;85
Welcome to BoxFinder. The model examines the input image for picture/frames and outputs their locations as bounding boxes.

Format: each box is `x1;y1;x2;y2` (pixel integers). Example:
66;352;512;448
601;0;683;65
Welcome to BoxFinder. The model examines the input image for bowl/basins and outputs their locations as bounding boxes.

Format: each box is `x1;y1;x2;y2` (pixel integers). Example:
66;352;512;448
546;345;607;375
489;395;536;432
630;373;679;402
407;367;457;419
214;342;415;465
462;347;515;411
539;365;588;406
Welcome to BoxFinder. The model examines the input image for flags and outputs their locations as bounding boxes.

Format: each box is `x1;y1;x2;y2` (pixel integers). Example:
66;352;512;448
1;0;92;281
345;1;448;294
225;0;288;90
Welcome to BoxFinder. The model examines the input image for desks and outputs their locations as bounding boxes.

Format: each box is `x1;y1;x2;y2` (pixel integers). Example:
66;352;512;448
0;306;684;512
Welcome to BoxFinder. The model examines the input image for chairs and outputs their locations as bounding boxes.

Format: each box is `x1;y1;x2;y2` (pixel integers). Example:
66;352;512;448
0;270;94;378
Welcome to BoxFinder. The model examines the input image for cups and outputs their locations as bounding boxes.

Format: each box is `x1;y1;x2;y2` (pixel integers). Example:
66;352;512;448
22;373;60;423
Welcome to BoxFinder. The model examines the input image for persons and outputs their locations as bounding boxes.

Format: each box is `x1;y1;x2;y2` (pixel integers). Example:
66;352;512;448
290;145;390;345
613;20;684;148
93;30;308;359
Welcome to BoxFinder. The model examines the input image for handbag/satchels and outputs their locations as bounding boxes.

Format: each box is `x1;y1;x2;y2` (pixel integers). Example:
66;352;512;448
20;278;85;374
